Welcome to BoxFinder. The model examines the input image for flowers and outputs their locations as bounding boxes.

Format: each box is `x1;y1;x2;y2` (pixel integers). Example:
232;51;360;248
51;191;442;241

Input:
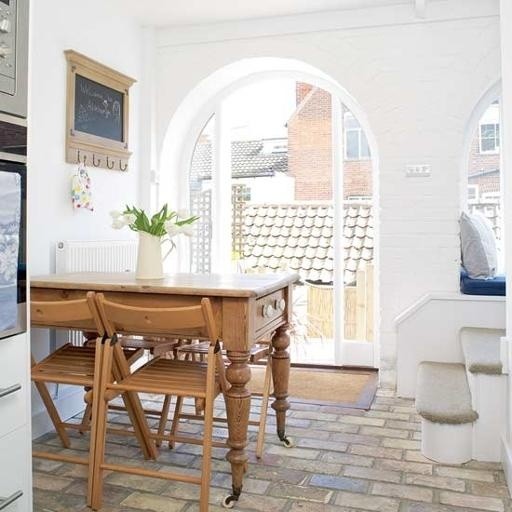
108;201;203;240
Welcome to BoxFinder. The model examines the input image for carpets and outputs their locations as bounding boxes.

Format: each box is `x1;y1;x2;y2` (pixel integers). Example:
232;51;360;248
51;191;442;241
244;364;380;412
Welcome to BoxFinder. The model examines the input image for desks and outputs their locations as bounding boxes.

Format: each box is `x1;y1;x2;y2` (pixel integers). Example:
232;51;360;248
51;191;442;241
29;271;303;509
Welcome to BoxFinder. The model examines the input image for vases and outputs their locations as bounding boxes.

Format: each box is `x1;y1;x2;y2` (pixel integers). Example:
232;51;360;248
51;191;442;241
132;229;175;281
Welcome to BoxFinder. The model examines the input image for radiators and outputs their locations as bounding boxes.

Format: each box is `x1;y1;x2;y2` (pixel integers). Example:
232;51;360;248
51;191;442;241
51;238;137;351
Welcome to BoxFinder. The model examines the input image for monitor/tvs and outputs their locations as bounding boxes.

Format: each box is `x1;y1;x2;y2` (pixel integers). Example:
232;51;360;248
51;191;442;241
0;160;29;343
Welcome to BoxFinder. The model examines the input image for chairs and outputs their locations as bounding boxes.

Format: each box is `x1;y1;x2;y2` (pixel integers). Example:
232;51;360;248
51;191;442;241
77;337;201;449
30;291;151;508
166;340;273;461
90;291;229;512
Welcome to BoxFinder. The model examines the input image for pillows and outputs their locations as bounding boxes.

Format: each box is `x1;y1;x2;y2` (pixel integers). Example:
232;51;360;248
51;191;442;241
458;205;501;281
